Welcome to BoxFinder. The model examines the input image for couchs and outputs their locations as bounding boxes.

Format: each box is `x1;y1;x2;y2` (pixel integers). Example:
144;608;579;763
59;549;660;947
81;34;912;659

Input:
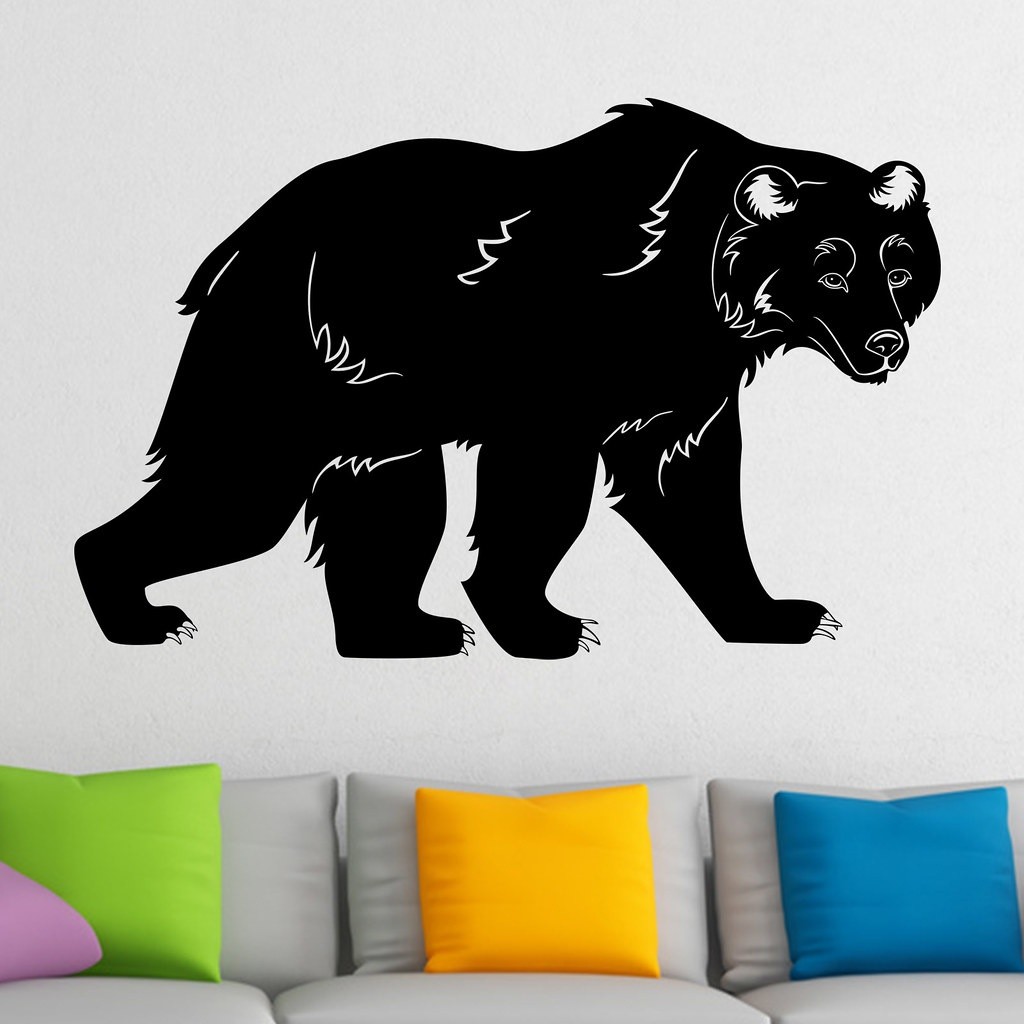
0;777;1024;1024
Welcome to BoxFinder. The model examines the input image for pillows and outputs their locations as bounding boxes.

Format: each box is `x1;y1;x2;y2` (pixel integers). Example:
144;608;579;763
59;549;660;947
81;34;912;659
0;762;224;984
0;860;103;985
774;785;1024;983
415;781;663;980
